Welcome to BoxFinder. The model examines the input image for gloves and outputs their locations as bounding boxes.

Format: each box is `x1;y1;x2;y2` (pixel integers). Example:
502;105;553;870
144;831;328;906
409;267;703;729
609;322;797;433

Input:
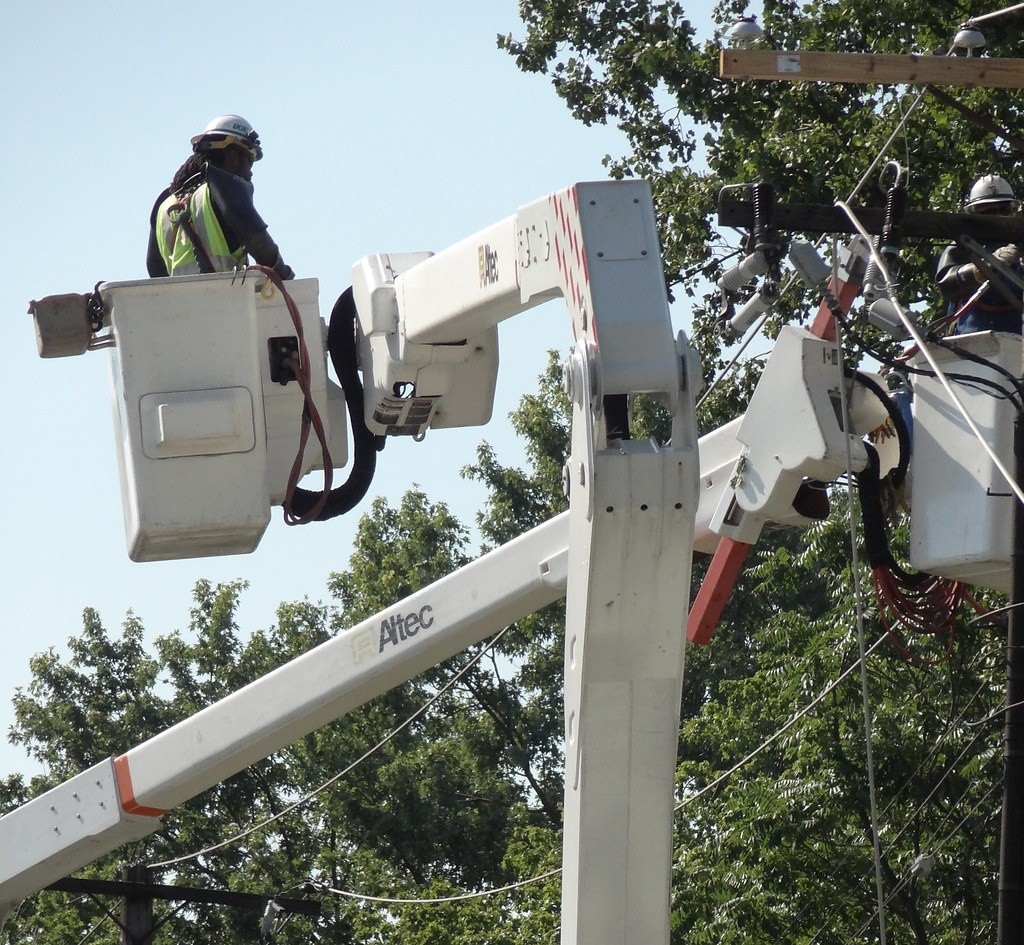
973;243;1021;284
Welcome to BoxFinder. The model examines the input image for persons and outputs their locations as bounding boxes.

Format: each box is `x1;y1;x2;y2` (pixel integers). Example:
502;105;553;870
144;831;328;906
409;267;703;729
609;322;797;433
148;115;296;280
936;174;1024;335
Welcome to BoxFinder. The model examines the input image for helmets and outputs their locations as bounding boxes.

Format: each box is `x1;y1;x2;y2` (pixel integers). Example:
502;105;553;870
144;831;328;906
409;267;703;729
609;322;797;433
190;114;264;162
963;173;1021;214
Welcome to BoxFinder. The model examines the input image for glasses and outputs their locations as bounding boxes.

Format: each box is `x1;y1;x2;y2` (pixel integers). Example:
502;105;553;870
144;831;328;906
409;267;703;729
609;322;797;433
970;206;1011;216
